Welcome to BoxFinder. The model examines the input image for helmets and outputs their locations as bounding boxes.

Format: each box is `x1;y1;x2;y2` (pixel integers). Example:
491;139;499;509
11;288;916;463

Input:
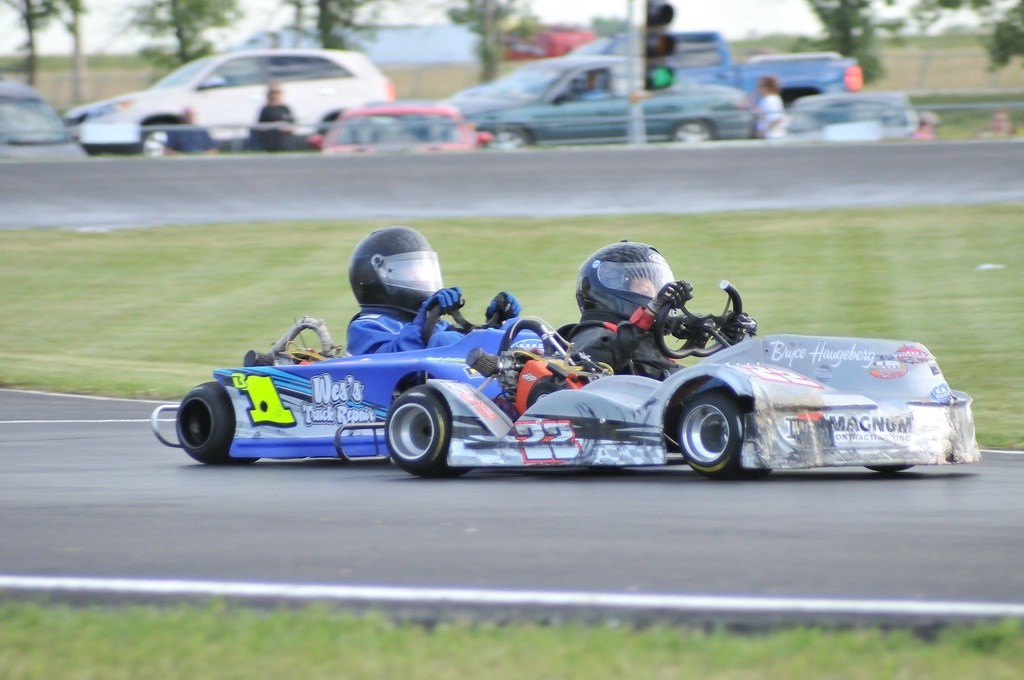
348;226;443;316
575;240;677;336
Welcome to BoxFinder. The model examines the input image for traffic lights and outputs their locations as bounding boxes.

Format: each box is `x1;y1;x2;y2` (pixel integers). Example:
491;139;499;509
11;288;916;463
646;0;674;91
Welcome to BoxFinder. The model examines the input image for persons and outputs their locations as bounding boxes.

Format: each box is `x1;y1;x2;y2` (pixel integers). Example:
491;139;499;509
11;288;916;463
254;83;303;150
975;109;1014;138
736;75;787;138
557;242;757;379
345;226;520;355
146;106;219;157
574;71;608;100
910;110;940;140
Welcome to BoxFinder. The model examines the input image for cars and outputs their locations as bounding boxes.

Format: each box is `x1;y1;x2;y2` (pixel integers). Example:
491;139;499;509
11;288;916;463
435;54;758;153
0;82;85;158
775;92;934;143
61;50;396;158
308;101;496;151
499;27;592;59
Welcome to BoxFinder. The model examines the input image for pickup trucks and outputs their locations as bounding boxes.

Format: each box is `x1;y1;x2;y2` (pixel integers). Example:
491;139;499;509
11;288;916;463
576;30;866;110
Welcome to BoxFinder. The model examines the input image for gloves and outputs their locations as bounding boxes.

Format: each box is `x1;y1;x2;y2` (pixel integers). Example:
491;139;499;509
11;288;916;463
484;292;520;327
724;312;748;341
647;281;693;316
414;287;465;325
